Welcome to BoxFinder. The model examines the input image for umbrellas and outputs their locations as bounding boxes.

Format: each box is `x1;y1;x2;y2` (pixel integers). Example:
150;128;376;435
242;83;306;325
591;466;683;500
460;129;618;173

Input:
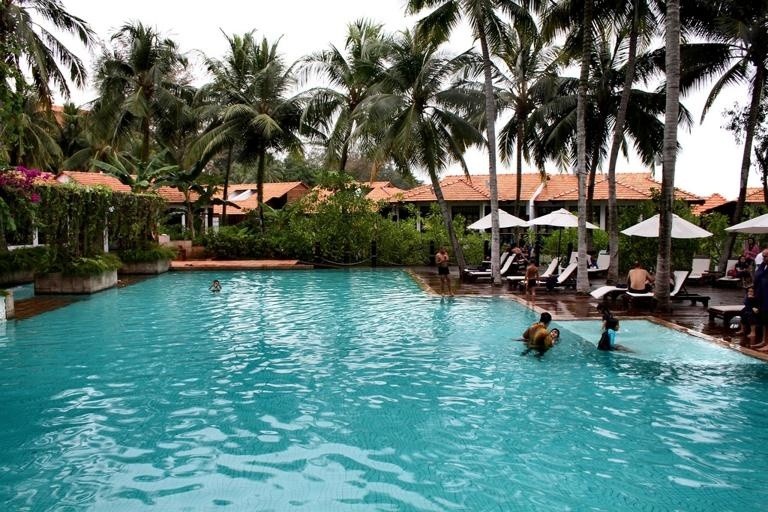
467;208;529;231
620;211;713;239
526;207;602;264
724;212;768;237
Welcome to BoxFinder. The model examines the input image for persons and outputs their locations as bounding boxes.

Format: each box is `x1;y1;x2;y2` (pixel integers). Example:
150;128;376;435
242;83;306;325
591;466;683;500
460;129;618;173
596;317;634;352
625;260;656;293
435;245;455;297
735;255;753;289
750;248;768;350
511;327;559;356
523;258;539;306
207;278;221;292
525;312;553;351
743;236;761;283
596;302;615;327
735;286;759;337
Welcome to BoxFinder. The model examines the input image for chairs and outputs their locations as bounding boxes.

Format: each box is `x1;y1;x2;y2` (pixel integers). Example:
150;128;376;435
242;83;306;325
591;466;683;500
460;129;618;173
461;249;611;293
587;254;758;332
